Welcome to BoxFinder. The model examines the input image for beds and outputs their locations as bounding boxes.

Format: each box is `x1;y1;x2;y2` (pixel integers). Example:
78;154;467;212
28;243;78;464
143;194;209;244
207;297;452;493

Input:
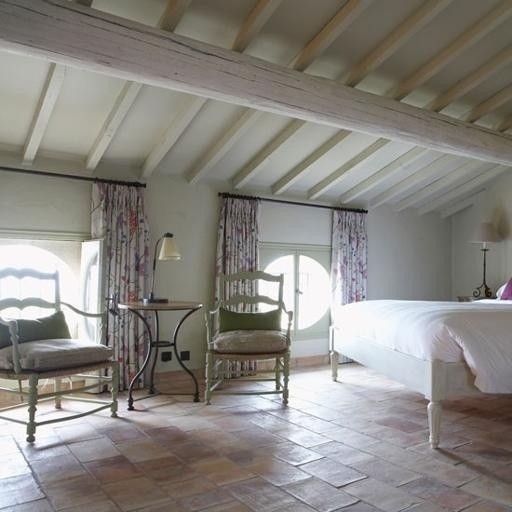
327;278;512;449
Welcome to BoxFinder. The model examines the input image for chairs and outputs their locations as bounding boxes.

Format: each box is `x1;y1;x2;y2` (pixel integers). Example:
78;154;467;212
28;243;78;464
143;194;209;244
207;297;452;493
203;269;293;406
0;268;119;444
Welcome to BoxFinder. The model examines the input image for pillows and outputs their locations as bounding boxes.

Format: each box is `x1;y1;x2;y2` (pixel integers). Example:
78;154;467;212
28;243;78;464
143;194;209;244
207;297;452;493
0;309;73;348
219;307;281;333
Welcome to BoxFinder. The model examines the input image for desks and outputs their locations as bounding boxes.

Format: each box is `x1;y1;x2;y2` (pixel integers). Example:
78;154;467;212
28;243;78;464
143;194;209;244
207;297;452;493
117;301;202;410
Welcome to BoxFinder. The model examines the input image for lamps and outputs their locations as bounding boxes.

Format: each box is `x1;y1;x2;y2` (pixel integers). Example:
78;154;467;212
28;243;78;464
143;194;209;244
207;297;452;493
143;232;182;304
466;222;502;297
